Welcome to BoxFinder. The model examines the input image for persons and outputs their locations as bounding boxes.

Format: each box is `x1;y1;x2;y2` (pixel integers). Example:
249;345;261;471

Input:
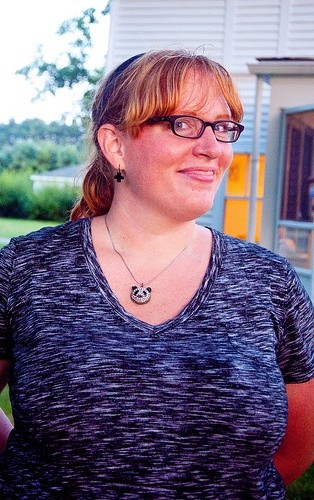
0;50;314;500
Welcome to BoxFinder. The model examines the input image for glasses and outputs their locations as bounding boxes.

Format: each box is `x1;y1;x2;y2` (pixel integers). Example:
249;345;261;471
141;114;245;144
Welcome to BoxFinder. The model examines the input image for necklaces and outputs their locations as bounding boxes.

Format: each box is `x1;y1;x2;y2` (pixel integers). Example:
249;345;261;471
102;209;191;305
